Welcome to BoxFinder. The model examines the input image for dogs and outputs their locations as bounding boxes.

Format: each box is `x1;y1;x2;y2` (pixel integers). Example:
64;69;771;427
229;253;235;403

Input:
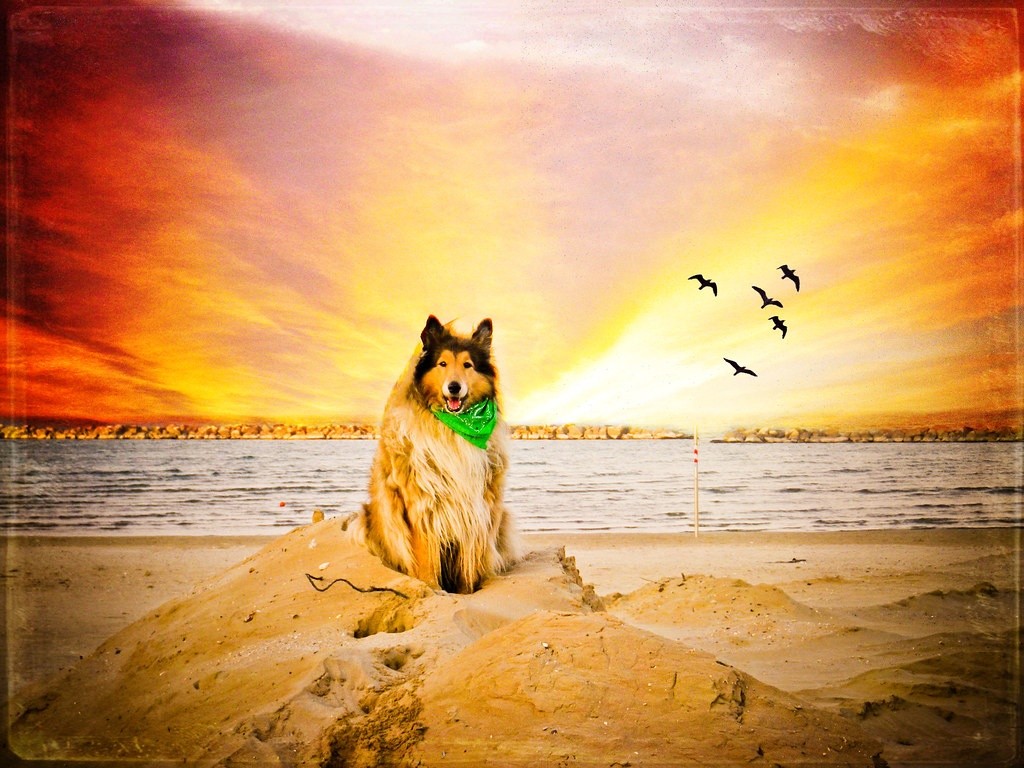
368;313;524;596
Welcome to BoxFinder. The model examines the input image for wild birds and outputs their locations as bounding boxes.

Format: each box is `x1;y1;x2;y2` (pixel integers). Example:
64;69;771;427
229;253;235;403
768;316;788;339
724;357;757;377
752;285;783;309
688;274;718;297
777;265;800;292
